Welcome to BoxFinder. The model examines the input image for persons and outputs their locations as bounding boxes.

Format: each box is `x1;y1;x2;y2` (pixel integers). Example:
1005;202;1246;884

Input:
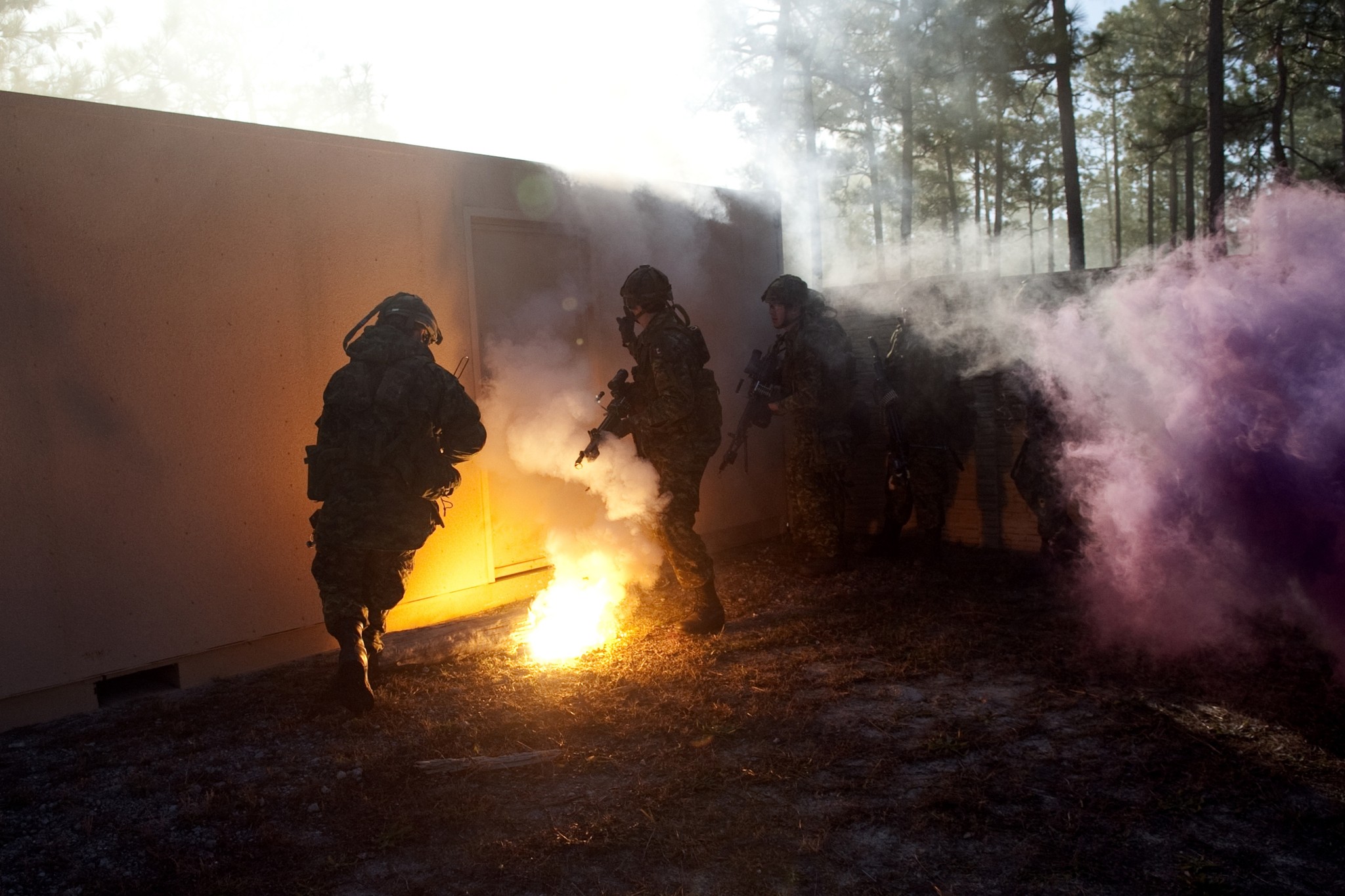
304;290;489;713
1009;277;1092;588
857;275;975;571
601;263;727;636
752;274;876;582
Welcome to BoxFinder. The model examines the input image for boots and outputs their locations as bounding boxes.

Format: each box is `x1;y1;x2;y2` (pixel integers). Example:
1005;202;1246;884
366;653;382;690
333;618;375;714
673;573;726;635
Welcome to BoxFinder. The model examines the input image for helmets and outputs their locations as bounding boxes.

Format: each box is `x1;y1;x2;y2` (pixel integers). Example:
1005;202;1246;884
620;264;673;302
761;274;809;303
378;293;444;346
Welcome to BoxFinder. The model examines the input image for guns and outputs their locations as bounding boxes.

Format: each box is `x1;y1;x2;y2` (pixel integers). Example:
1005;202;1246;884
716;332;790;475
574;364;644;470
866;333;913;470
